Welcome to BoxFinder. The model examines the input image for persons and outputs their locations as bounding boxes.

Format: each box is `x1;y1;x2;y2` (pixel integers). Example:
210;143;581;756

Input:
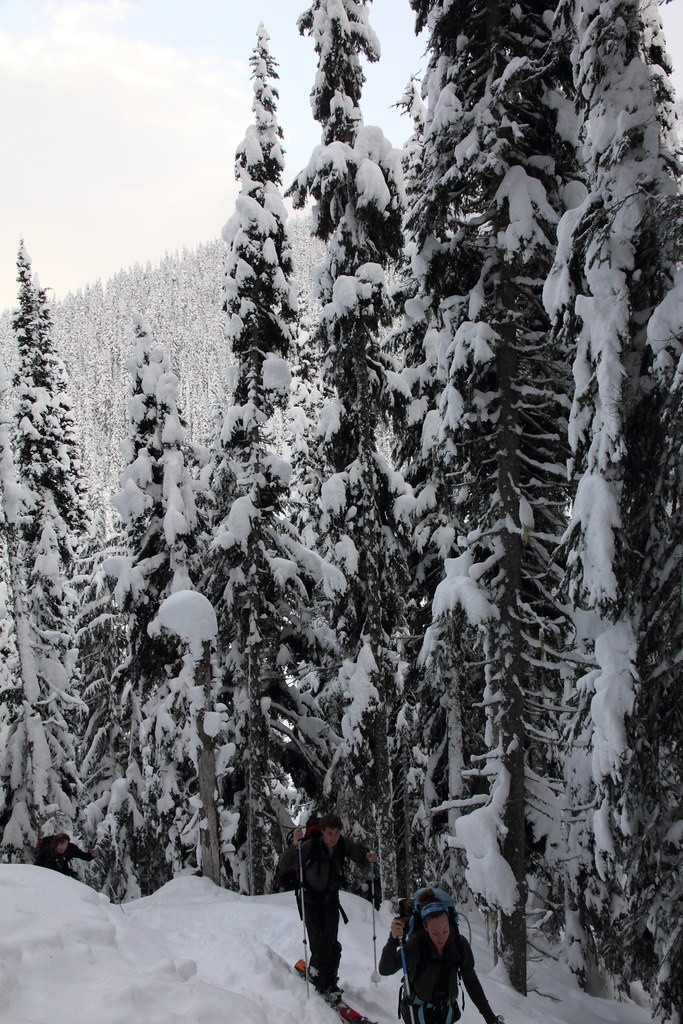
378;888;506;1024
278;812;378;1006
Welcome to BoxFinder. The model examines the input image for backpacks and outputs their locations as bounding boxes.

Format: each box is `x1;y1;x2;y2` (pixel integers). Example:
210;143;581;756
406;887;458;938
296;815;320;843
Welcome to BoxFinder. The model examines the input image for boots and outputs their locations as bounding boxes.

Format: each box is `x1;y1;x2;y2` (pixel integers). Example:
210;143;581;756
308;958;331;995
325;953;343;1005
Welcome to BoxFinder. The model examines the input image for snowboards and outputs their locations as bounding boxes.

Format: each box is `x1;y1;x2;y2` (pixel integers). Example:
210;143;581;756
296;958;372;1024
